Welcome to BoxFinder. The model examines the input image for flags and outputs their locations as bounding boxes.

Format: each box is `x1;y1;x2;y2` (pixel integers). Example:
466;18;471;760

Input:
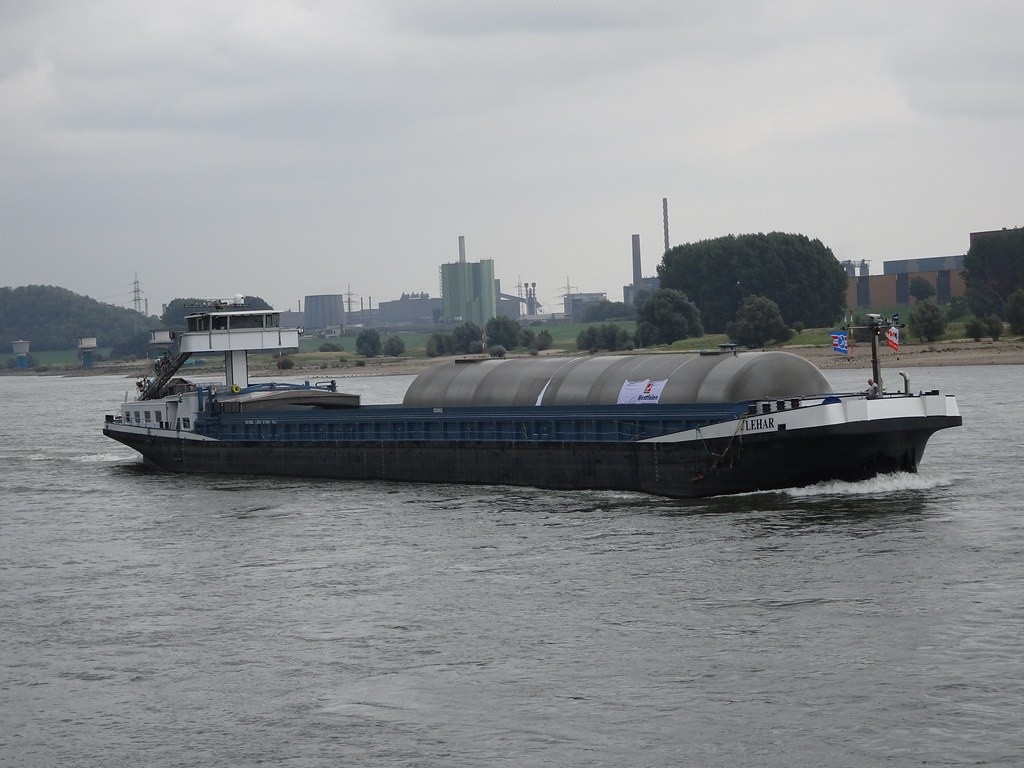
829;331;848;354
885;327;899;351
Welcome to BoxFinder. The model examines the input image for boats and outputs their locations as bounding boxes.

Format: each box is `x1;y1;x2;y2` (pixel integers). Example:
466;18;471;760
102;295;963;497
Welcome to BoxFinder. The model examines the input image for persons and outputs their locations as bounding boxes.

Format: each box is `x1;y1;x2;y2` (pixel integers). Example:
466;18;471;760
865;379;880;400
141;350;172;391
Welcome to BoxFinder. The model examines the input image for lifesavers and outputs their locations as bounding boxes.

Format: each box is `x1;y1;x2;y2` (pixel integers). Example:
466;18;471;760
232;385;241;393
